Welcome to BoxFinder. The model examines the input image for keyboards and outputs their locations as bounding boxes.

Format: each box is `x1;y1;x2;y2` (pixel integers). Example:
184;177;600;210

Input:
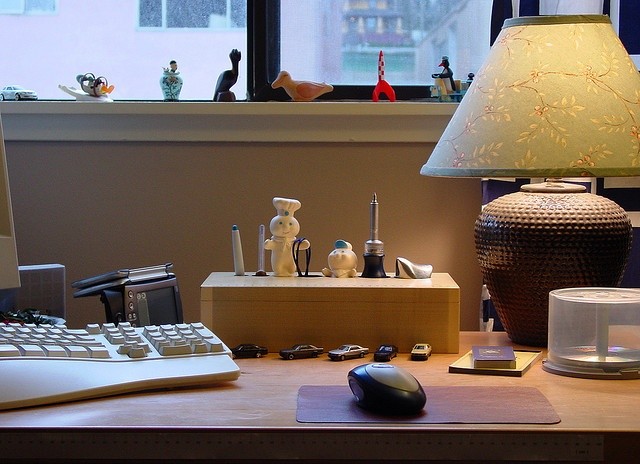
0;318;241;415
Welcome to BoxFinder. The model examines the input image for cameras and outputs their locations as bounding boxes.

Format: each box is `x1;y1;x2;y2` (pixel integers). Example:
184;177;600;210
69;261;183;327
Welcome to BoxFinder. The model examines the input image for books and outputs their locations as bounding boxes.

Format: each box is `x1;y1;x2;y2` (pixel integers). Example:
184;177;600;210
472;346;516;370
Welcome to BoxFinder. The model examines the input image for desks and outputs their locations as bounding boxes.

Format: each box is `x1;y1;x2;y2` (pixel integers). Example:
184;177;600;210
0;331;640;464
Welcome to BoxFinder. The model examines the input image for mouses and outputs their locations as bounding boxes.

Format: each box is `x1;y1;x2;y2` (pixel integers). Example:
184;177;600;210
346;363;428;421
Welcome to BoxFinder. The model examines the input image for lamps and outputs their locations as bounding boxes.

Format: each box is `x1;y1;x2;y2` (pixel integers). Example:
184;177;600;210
419;13;640;345
